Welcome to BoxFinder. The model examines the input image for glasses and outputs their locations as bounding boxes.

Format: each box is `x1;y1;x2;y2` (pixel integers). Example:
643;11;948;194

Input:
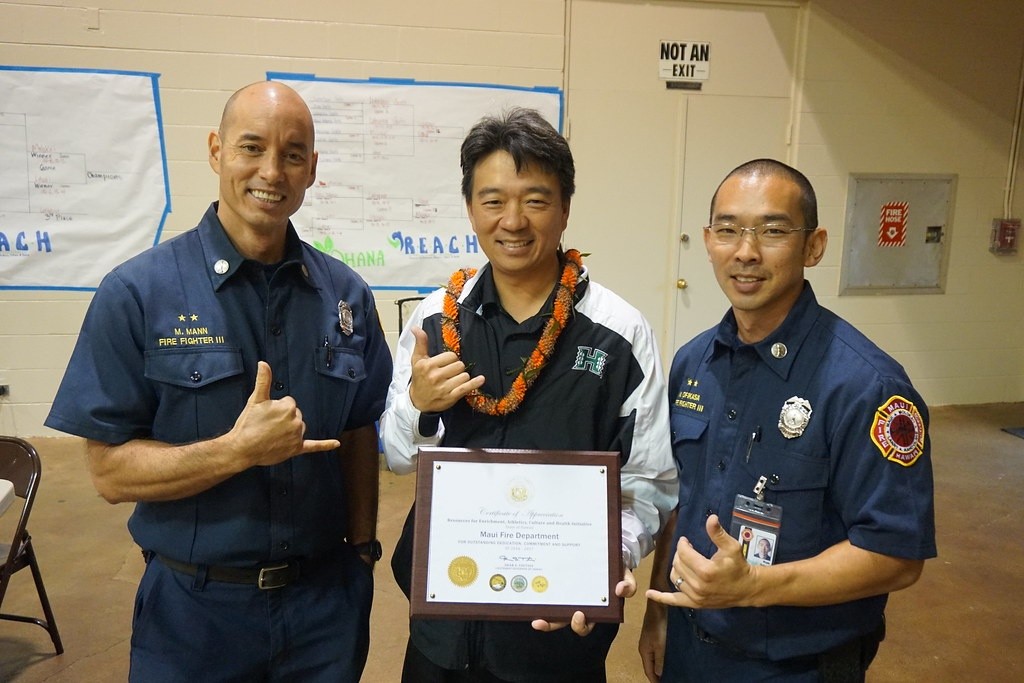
705;223;814;246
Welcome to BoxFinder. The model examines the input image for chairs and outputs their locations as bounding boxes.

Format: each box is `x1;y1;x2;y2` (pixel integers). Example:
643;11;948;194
0;436;63;654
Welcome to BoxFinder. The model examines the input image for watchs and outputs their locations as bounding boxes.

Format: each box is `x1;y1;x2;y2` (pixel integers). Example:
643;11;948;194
357;540;382;560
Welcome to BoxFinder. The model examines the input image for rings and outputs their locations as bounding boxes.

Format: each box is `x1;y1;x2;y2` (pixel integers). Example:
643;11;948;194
674;577;683;591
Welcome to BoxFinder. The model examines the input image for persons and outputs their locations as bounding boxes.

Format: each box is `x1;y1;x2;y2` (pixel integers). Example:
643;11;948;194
379;105;679;683
44;80;392;683
753;539;771;560
637;158;939;683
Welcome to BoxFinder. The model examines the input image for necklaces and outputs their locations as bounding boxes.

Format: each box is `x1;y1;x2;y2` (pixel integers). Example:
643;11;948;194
442;249;582;414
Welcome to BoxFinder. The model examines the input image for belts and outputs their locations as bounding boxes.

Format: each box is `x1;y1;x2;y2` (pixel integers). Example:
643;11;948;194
153;541;346;591
689;621;746;657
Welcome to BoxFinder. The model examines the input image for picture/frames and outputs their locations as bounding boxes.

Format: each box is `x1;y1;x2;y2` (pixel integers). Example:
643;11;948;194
408;446;625;622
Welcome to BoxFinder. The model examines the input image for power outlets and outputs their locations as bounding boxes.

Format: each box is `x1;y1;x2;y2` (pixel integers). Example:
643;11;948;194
0;385;9;395
